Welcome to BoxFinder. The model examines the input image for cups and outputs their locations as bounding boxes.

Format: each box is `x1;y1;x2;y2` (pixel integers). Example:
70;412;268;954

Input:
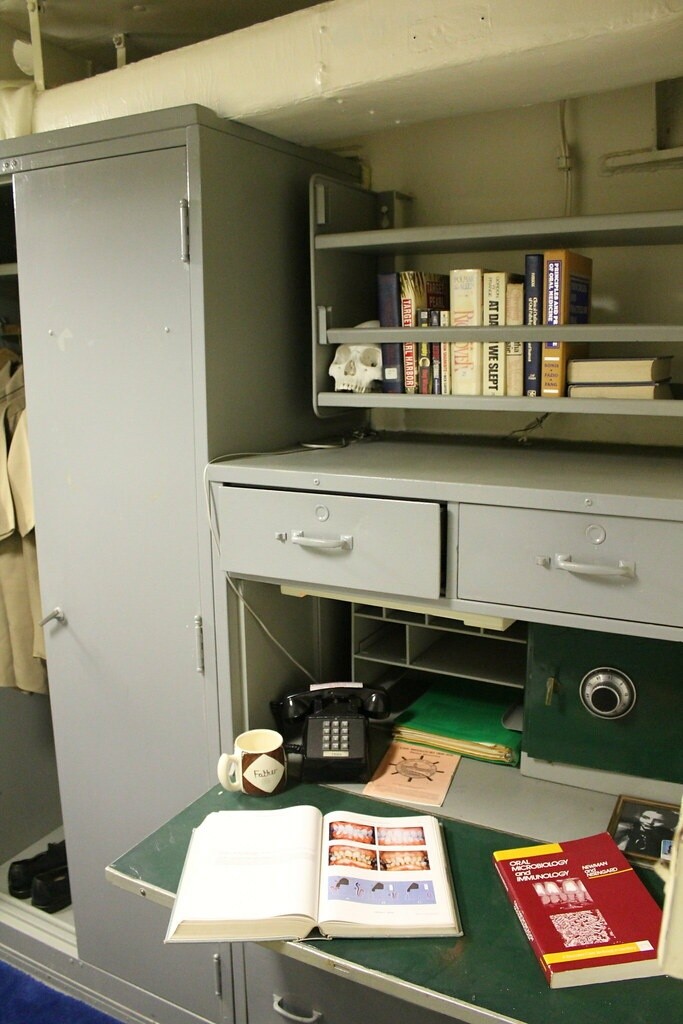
217;728;289;797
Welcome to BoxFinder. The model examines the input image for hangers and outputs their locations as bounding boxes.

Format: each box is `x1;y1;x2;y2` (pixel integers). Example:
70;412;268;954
0;316;21;377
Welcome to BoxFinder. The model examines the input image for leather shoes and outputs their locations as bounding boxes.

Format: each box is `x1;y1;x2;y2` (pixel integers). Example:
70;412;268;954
8;839;72;914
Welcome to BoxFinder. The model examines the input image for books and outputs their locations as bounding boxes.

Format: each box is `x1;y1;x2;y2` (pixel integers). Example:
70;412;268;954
392;678;523;767
163;804;465;944
493;831;669;989
362;742;462;807
378;248;675;400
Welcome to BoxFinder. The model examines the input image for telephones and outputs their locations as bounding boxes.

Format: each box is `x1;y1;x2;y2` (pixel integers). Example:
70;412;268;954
269;681;391;785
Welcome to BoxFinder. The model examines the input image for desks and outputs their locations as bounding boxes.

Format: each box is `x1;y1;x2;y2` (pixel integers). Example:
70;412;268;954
106;743;683;1024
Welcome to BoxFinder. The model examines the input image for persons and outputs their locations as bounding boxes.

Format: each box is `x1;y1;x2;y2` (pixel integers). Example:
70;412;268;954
615;805;679;858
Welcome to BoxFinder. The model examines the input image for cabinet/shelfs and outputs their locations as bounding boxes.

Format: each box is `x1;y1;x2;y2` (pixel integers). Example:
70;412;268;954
12;144;308;1024
211;438;683;644
313;207;683;419
352;603;524;697
